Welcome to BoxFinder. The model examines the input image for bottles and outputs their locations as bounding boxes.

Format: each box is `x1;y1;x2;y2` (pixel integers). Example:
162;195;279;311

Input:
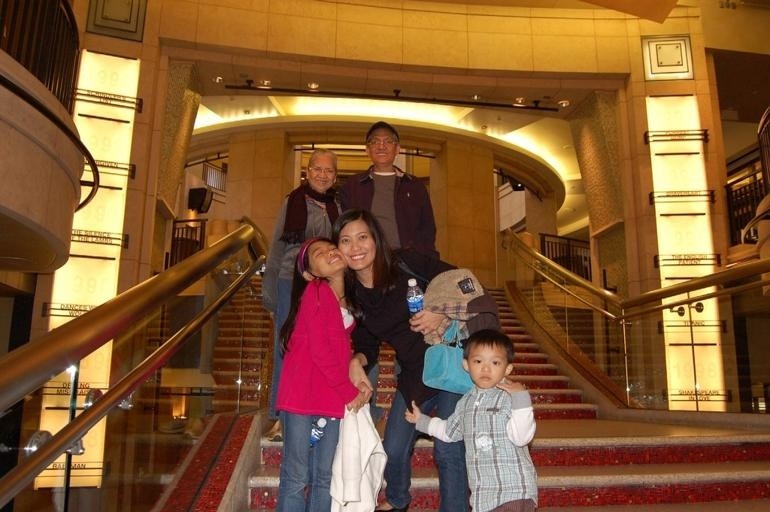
310;417;327;449
405;279;426;321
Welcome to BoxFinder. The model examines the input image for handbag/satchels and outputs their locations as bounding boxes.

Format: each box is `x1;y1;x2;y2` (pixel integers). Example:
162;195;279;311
421;343;478;395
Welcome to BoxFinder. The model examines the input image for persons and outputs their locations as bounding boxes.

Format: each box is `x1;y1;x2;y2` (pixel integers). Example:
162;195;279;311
262;150;344;441
275;236;374;512
404;329;539;512
335;209;498;511
337;118;437;423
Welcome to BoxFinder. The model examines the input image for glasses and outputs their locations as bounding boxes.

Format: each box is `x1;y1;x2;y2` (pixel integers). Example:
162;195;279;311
367;140;400;146
307;165;336;175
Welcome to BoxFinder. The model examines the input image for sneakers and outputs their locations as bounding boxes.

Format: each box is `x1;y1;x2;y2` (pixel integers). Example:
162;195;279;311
267;417;283;443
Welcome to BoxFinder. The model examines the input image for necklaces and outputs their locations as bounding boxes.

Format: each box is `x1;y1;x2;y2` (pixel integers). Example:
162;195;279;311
337;293;345;302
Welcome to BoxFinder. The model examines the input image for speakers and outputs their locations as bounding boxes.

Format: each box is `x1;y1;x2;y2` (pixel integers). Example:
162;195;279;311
188;188;213;213
